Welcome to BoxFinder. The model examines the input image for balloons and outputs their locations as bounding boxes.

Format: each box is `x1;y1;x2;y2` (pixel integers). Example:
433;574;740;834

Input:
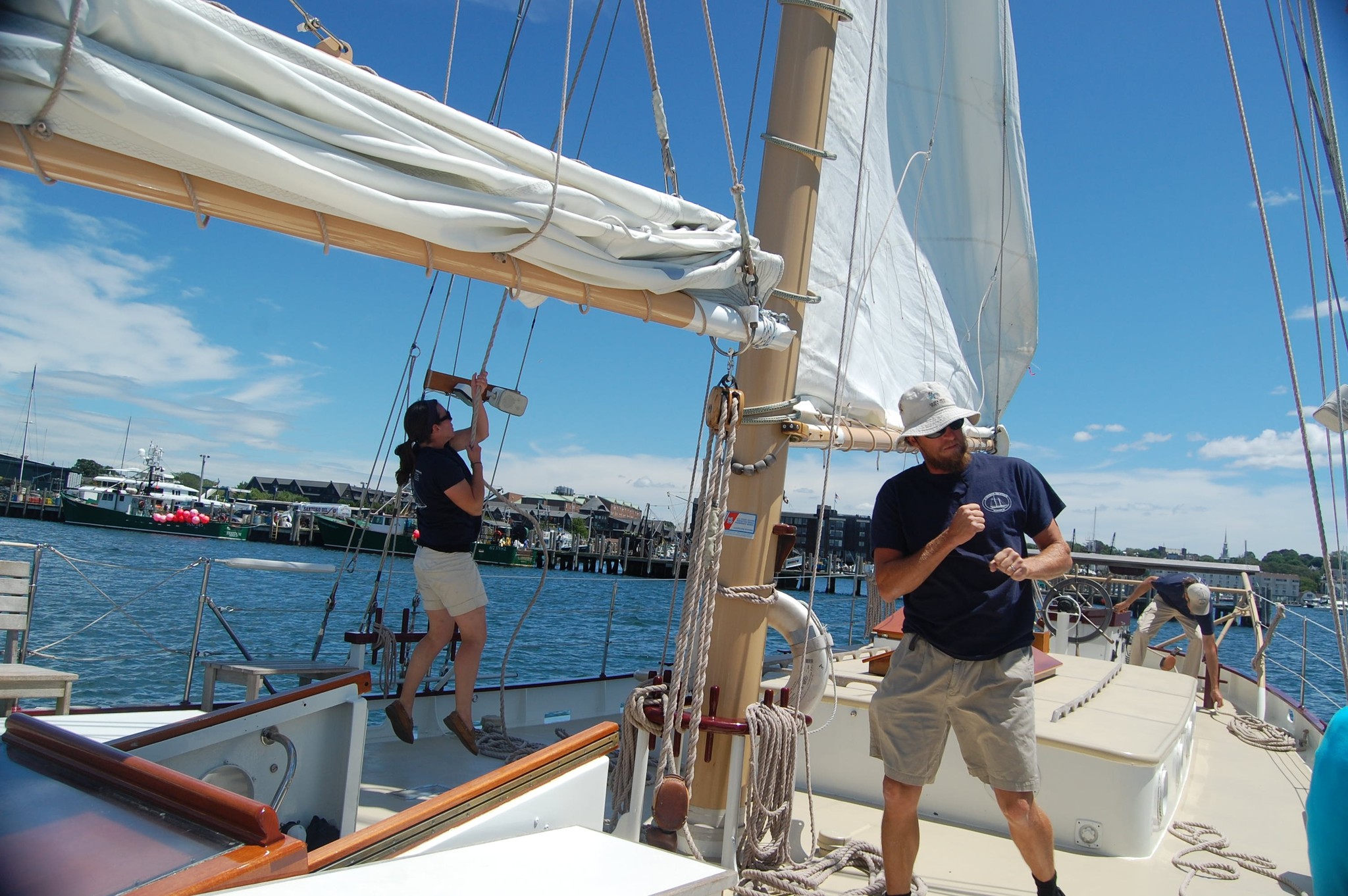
155;509;209;524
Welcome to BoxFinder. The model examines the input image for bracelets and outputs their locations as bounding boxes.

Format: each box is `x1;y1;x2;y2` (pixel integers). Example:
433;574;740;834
471;462;482;469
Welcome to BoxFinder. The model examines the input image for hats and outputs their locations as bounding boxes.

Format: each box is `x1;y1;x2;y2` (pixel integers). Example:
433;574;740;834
894;382;980;451
1187;583;1210;615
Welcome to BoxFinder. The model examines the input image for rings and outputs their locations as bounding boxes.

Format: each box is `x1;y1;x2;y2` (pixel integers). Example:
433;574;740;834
1010;565;1016;572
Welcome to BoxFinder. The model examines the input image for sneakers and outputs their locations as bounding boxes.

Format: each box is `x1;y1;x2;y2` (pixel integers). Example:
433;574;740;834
386;700;415;744
443;711;478;755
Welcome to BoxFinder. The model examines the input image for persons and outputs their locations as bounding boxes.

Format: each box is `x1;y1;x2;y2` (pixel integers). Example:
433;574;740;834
255;509;279;526
556;533;612;555
869;381;1073;896
385;371;489;756
805;556;867;575
492;528;540;549
200;510;228;523
55;492;61;505
163;504;168;515
1113;572;1224;708
1305;706;1348;896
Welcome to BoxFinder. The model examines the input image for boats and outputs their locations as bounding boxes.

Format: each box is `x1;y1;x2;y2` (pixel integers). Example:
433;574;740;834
60;436;249;541
307;462;541;570
1303;594;1348;616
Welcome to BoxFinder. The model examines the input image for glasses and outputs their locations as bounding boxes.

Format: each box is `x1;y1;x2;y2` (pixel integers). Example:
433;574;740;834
435;411;452;424
924;416;964;438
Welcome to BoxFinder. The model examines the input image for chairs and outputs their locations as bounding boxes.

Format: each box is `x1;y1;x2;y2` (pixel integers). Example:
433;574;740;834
0;541;79;716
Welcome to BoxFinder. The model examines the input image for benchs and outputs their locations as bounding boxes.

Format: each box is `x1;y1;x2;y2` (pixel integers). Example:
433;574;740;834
199;656;361;713
